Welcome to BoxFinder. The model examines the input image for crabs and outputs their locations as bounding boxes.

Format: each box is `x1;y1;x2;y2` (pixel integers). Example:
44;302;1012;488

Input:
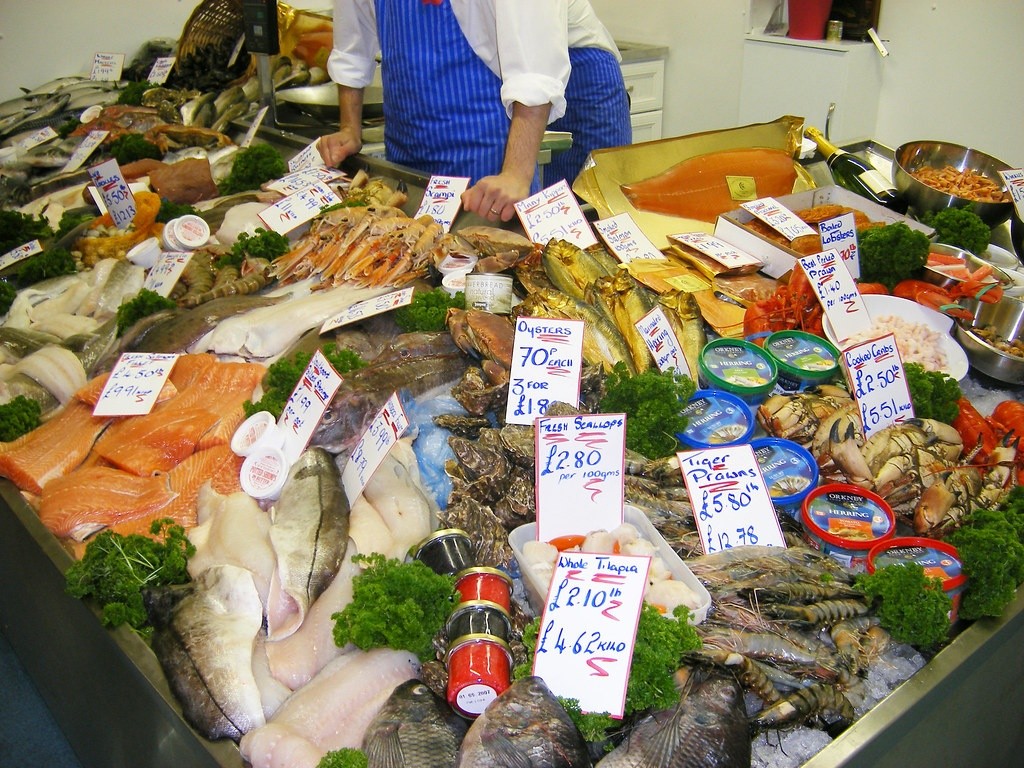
445;226;535;387
757;384;1020;539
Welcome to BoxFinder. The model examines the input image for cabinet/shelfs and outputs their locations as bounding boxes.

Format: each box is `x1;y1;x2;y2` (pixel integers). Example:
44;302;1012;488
734;32;886;145
615;40;670;146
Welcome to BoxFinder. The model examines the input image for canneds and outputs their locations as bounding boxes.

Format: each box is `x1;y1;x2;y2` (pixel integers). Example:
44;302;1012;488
464;272;514;315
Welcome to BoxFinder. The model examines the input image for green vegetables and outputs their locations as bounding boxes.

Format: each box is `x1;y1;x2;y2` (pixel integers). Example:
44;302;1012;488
241;340;367;420
855;207;990;286
392;288;466;333
0;76;375;332
856;480;1024;648
900;361;962;427
595;361;698;461
331;549;702;752
0;394;197;641
315;747;369;768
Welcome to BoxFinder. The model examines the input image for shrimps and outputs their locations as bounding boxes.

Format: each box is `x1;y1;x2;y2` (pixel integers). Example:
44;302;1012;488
624;448;890;740
168;205;475;309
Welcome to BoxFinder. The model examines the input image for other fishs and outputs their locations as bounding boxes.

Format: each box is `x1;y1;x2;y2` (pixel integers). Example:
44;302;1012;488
0;237;754;768
0;57;328;167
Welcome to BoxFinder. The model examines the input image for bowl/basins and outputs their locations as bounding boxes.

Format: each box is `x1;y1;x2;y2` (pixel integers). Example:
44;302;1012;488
891;139;1016;231
952;295;1024;387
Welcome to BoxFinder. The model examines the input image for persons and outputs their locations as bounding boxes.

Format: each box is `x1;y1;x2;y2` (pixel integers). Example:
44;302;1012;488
315;1;632;222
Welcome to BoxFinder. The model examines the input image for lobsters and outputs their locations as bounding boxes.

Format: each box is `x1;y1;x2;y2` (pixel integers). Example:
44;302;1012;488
715;263;1024;486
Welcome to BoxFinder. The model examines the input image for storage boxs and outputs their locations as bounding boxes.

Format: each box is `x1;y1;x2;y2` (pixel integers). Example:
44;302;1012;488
507;506;712;626
711;182;941;282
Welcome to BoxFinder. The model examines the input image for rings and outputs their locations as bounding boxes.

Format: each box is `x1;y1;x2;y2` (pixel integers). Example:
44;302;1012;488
490;208;500;216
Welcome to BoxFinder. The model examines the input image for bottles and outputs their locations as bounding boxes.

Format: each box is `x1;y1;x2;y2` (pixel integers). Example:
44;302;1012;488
803;125;909;215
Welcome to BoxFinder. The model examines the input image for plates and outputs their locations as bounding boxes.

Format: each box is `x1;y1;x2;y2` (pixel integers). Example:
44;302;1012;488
822;292;970;383
